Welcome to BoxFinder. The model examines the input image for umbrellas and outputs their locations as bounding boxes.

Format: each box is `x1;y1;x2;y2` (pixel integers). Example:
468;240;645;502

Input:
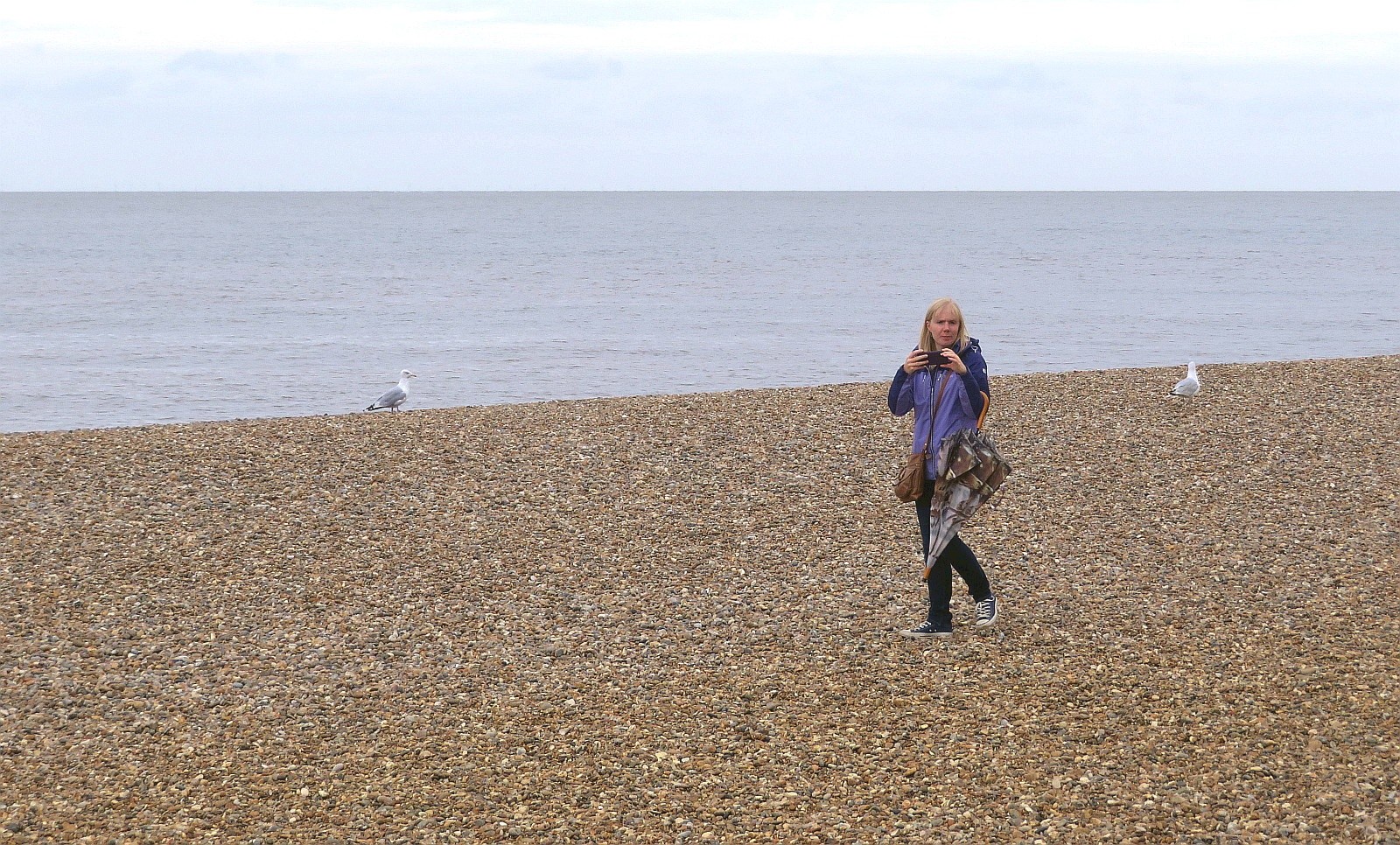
922;391;1013;583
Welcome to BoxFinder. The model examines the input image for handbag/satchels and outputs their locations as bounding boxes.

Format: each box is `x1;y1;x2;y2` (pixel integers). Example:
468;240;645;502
895;452;924;503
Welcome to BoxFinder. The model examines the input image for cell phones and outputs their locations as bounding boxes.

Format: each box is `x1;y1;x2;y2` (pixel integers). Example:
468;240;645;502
916;350;951;365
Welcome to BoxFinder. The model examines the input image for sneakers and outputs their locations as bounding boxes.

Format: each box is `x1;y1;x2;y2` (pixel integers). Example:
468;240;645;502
975;596;998;630
899;621;954;640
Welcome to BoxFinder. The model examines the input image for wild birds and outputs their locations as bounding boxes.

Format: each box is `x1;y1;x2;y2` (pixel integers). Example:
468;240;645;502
362;368;417;415
1167;360;1201;403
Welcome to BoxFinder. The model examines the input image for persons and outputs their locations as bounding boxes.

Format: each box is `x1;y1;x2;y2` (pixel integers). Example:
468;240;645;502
886;297;999;638
